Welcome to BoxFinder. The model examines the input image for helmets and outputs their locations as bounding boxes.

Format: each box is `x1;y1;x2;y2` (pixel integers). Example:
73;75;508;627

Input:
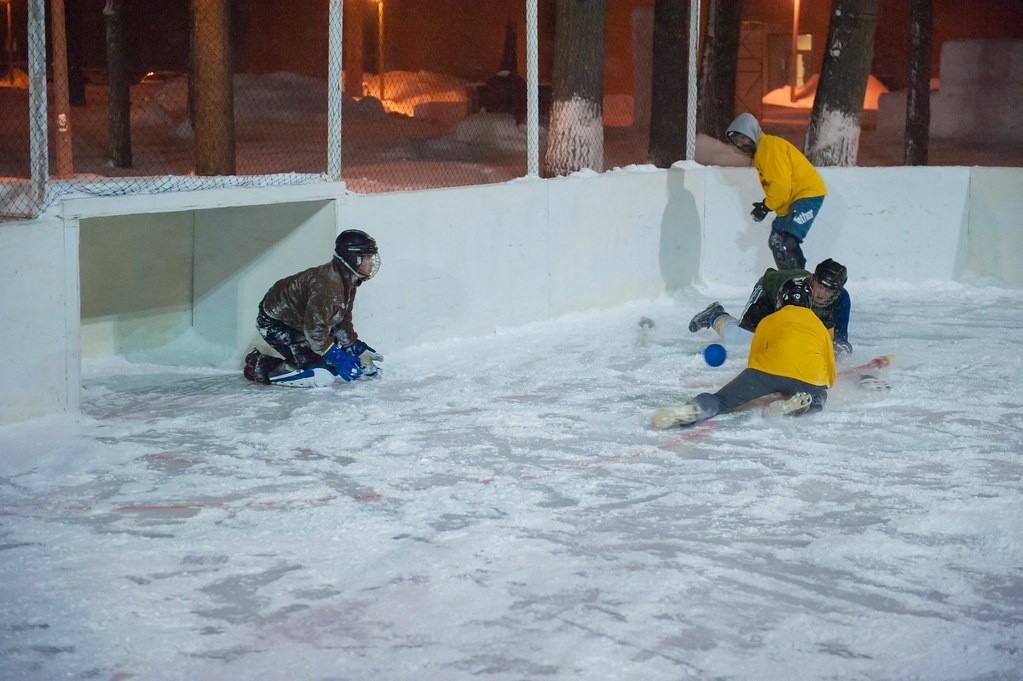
775;277;811;311
334;230;381;282
808;259;848;307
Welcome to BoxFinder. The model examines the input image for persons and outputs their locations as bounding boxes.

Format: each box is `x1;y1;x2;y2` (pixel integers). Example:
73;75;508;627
724;112;828;270
243;229;385;390
687;257;853;364
649;305;835;429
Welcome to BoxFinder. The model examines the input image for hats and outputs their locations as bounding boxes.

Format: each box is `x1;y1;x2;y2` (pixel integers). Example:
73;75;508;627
727;131;742;142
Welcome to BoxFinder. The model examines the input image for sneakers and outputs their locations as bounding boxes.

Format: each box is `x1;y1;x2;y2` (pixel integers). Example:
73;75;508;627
244;348;270;385
689;302;725;333
762;392;812;418
651;402;701;428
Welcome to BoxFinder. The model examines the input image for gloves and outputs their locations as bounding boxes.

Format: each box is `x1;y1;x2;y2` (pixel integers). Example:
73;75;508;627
834;340;853;361
321;341;366;382
343;339;384;380
750;198;770;223
